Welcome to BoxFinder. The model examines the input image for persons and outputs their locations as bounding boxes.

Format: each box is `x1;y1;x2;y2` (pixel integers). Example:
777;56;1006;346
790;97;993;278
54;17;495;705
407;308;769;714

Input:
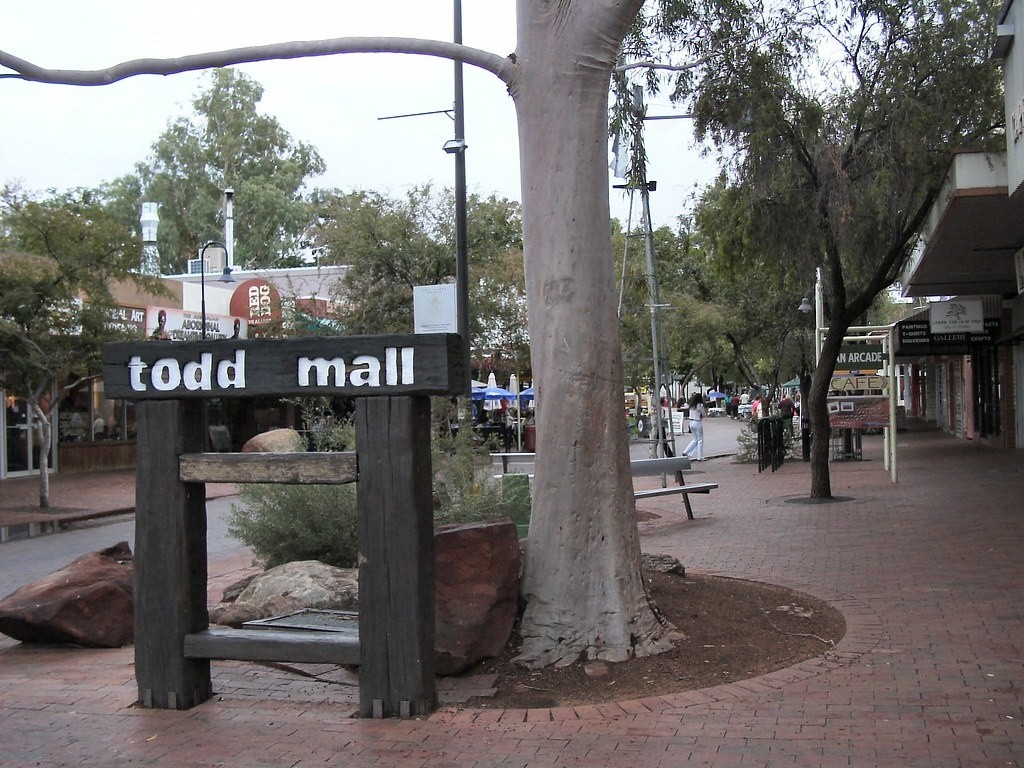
682;393;707;462
450;397;535;453
656;397;676;457
778;394;799;436
113;426;123;440
660;391;710;408
94;414;105;440
127;425;137;439
725;390;763;420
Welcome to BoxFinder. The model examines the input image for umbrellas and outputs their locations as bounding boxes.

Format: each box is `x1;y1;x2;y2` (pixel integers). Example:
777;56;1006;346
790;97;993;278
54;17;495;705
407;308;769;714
472;372;534;421
707;392;728;398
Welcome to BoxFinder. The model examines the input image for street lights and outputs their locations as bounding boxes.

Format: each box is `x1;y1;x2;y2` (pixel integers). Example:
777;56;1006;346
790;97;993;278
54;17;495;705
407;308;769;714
201;242;238;342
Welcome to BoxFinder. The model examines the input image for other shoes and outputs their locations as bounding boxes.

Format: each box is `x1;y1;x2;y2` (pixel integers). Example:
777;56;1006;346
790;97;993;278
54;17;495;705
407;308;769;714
698;459;707;461
682;452;688;457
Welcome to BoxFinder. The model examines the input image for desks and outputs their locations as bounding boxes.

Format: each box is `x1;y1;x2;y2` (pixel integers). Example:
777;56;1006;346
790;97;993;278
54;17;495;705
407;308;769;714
525;425;536;452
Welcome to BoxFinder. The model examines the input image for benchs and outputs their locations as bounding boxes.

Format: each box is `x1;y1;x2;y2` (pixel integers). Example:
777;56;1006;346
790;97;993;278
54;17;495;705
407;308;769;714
631;455;719;520
488;452;535;480
